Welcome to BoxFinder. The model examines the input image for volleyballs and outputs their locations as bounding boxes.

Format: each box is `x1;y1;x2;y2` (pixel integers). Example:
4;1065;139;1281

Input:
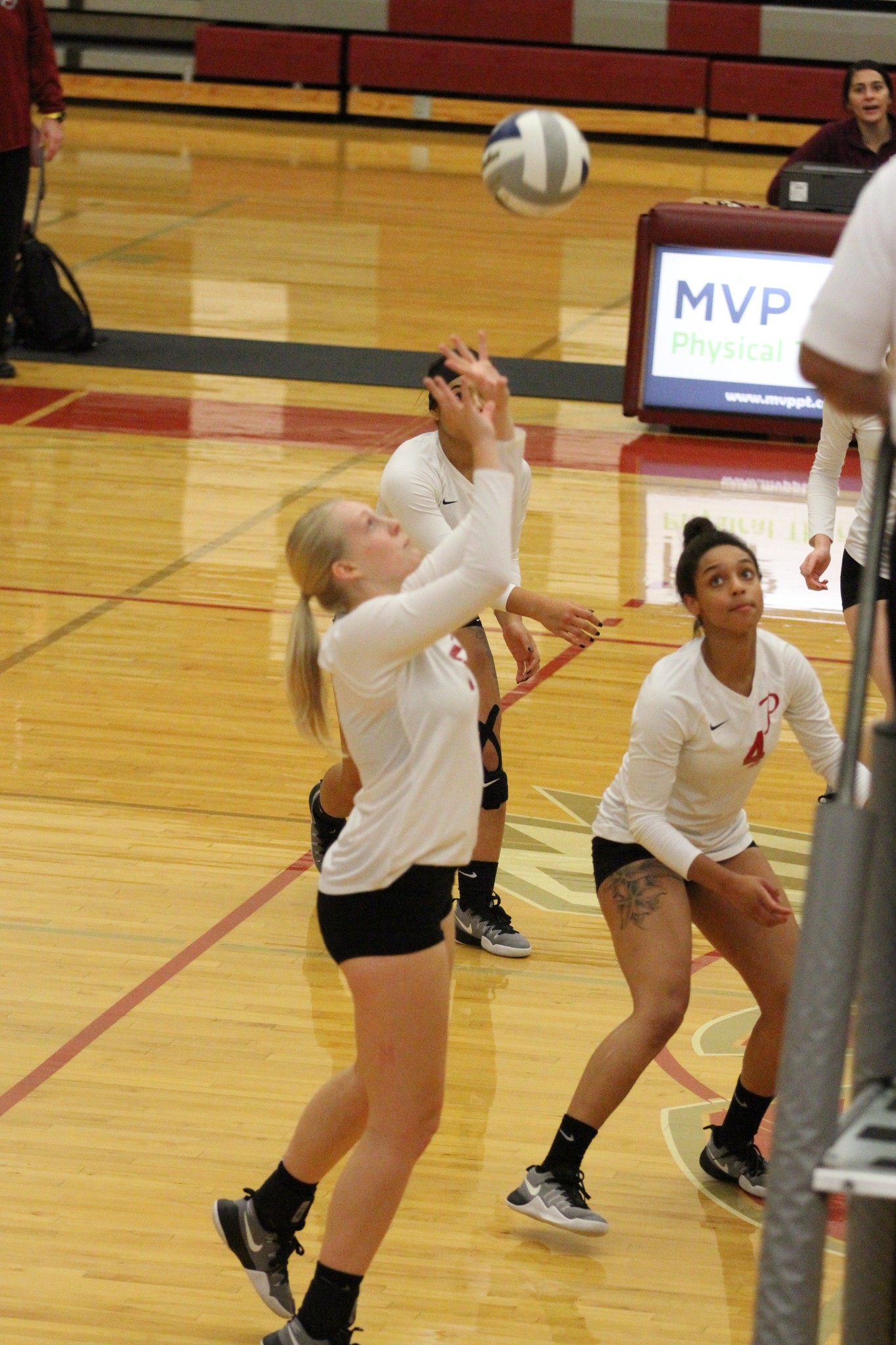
483;109;592;220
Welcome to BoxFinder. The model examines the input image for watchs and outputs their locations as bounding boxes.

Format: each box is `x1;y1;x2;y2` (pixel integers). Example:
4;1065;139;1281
46;111;65;123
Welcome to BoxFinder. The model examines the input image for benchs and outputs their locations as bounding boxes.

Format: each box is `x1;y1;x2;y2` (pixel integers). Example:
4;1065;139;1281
46;0;896;146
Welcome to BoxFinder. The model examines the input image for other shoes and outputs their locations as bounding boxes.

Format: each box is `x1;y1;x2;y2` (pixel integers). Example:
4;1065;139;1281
0;358;18;378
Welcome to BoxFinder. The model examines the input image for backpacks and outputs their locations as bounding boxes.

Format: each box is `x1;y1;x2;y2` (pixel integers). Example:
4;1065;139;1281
10;223;98;354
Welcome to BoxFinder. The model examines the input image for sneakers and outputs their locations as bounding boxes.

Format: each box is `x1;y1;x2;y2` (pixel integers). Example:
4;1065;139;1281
450;889;532;957
700;1124;770;1201
506;1164;608;1236
261;1315;364;1345
210;1187;307;1320
308;779;347;874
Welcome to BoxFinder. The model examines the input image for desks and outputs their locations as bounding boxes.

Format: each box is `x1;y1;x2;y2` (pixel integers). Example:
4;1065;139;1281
622;202;850;437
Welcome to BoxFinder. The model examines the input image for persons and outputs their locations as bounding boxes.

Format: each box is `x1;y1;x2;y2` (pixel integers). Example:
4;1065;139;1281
209;61;895;1345
1;0;69;376
